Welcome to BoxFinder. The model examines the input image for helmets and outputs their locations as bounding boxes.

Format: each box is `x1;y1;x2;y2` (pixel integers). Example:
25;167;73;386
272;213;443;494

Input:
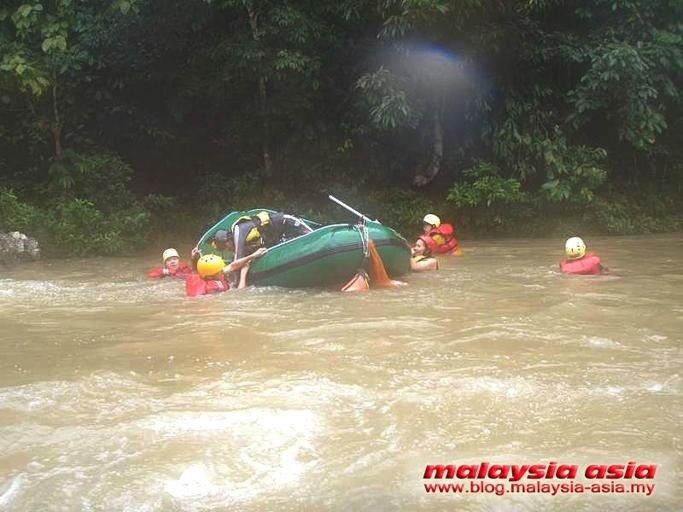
422;212;441;228
162;247;180;263
210;229;229;252
196;254;226;279
564;236;588;262
416;235;437;256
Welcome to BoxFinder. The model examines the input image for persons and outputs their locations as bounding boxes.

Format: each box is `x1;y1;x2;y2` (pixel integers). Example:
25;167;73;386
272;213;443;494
185;246;268;296
558;236;607;275
407;234;441;272
422;214;463;257
143;247;199;280
211;210;305;287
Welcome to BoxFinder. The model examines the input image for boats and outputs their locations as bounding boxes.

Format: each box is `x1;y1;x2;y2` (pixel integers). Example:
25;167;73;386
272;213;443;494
195;205;412;286
